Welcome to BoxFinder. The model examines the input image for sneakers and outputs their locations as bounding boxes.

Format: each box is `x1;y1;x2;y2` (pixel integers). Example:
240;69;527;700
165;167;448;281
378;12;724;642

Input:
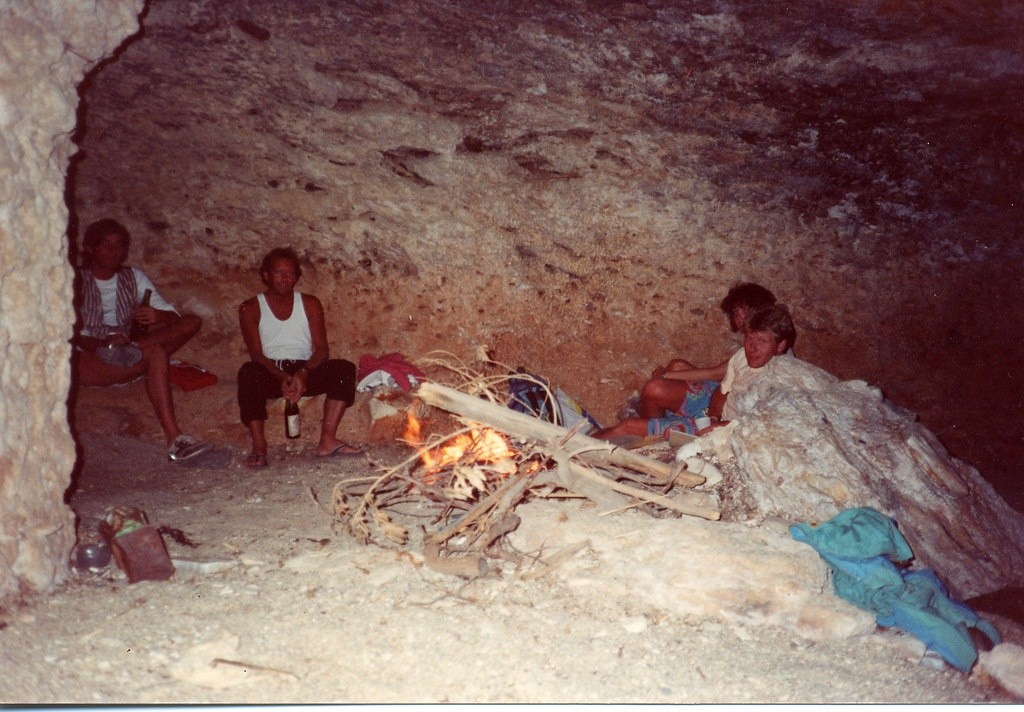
99;344;142;367
170;436;212;461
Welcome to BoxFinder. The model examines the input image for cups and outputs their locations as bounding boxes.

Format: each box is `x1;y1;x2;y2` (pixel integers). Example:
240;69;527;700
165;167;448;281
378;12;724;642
694;416;718;430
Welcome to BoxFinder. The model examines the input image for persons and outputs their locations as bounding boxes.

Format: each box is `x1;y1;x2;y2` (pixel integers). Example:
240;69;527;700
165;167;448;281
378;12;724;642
71;218;214;461
588;280;797;440
237;247;366;467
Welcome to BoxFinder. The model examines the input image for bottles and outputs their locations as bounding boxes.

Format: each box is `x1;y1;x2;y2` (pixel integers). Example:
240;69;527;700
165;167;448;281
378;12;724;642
128;288;151;343
284;376;301;439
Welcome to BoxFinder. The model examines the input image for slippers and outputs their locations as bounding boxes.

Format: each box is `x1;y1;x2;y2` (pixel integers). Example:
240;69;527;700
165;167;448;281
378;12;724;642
246;451;270;470
317;442;364;456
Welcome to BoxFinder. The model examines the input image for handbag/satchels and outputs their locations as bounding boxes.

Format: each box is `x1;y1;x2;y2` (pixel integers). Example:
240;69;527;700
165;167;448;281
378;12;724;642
506;367;603;434
